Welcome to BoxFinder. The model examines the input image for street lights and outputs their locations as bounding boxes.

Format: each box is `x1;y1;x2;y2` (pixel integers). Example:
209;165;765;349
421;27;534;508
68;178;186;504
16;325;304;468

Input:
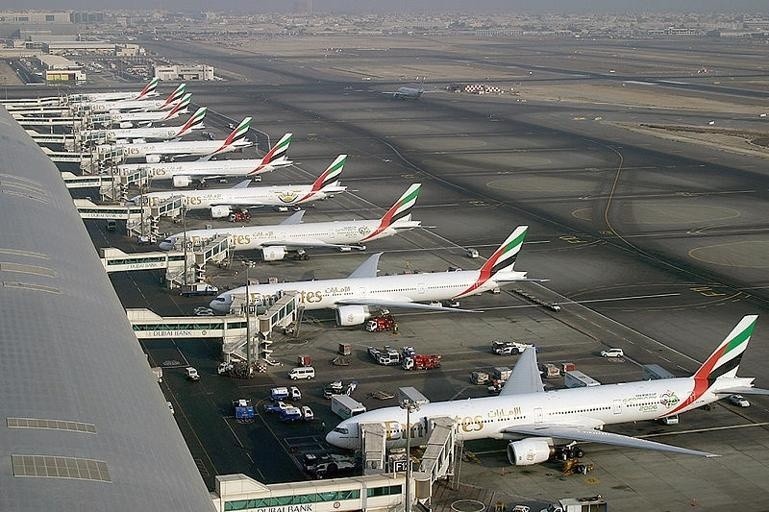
234;268;250;362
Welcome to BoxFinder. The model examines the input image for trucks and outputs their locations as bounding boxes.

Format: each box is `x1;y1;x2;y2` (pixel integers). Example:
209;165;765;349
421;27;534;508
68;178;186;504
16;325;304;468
229;213;251;224
540;494;608;512
564;370;600;388
151;366;163;385
398;386;429;406
267;276;278;284
280;404;314;424
106;219;116;233
642;362;677;381
217;362;255;379
249;277;259;285
331;395;367;422
270;386;302;403
264;401;294;416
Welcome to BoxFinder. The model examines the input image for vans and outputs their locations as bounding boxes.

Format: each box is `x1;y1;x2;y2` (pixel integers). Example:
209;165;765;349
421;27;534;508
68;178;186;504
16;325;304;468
468;248;479;259
288;367;315;381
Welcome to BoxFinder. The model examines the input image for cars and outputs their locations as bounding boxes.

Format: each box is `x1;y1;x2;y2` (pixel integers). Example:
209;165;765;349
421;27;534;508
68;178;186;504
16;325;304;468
86;197;92;201
729;394;750;410
193;306;215;317
166;401;174;415
512;505;531;512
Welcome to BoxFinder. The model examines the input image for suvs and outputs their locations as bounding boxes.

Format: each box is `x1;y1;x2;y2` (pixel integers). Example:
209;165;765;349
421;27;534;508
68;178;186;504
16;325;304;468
447;266;462;273
547;302;560;312
663;413;680;425
184;366;200;383
601;345;623;359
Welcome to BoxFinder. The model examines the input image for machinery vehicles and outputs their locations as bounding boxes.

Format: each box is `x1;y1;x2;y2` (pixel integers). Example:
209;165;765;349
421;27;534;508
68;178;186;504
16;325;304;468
366;314;395;333
322;381;359;399
385;346;400;365
304;453;346;473
316;461;355;479
401;353;442;371
489;338;541;355
231;399;256;427
401;346;415;359
367;343;391;366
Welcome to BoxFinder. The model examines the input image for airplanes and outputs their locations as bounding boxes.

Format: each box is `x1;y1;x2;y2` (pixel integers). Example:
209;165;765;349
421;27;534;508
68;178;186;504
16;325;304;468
129;154;349;212
159;183;425;260
58;73;294;186
211;226;533;325
379;76;450;101
324;314;767;460
